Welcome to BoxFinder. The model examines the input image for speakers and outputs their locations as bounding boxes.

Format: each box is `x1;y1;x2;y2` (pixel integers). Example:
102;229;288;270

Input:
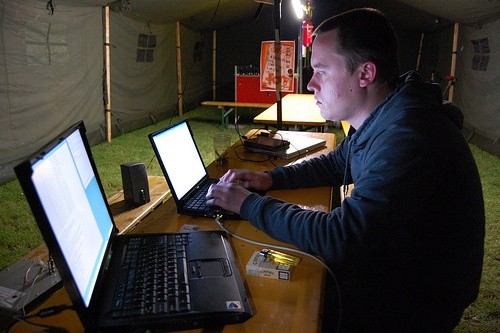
119;162;151;206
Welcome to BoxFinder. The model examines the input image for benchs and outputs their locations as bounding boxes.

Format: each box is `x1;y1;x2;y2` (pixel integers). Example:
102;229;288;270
23;176;167;266
201;101;273;130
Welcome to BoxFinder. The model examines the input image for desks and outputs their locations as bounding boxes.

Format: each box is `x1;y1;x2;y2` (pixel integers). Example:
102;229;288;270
233;73;298;120
253;93;327;133
6;128;338;333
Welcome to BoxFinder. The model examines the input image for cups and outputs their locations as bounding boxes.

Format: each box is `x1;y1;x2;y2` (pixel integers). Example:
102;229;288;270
212;134;232;172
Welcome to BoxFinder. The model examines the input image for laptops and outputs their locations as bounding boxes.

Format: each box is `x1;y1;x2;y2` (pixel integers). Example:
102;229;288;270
14;120;254;333
243;135;326;159
148;119;265;221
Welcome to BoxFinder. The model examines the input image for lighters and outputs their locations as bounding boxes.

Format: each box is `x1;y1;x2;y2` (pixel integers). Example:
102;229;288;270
260;248;301;266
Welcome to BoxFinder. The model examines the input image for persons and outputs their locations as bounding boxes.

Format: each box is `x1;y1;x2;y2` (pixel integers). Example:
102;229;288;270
205;8;486;333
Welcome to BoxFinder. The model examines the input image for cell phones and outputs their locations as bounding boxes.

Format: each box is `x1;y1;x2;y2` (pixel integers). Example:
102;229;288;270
244;136;290;149
